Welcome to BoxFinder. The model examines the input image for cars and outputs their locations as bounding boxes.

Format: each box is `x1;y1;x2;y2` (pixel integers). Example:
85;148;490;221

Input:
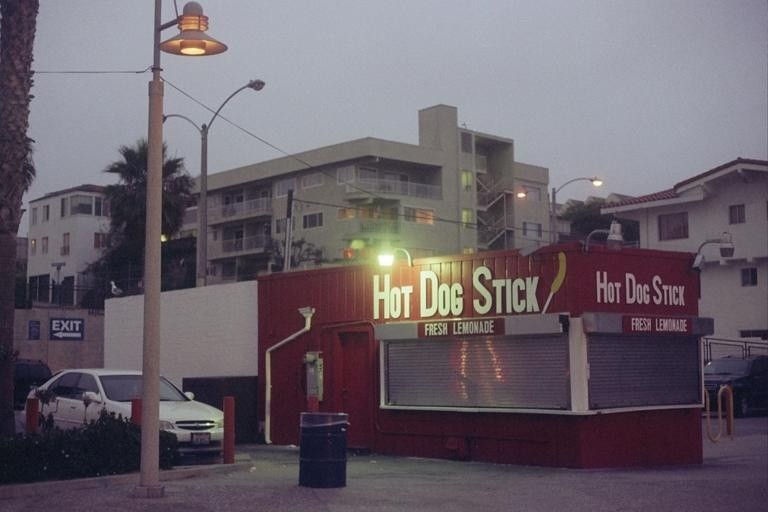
703;353;766;414
24;370;224;457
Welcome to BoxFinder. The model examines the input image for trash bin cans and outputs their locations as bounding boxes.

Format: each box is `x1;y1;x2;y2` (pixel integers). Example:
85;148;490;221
298;413;349;488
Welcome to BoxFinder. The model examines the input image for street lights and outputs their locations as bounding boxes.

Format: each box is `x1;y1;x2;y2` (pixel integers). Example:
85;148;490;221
551;176;603;245
164;79;269;288
138;2;229;497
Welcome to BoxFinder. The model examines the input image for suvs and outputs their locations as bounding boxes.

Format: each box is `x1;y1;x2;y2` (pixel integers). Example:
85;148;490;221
13;359;51;411
